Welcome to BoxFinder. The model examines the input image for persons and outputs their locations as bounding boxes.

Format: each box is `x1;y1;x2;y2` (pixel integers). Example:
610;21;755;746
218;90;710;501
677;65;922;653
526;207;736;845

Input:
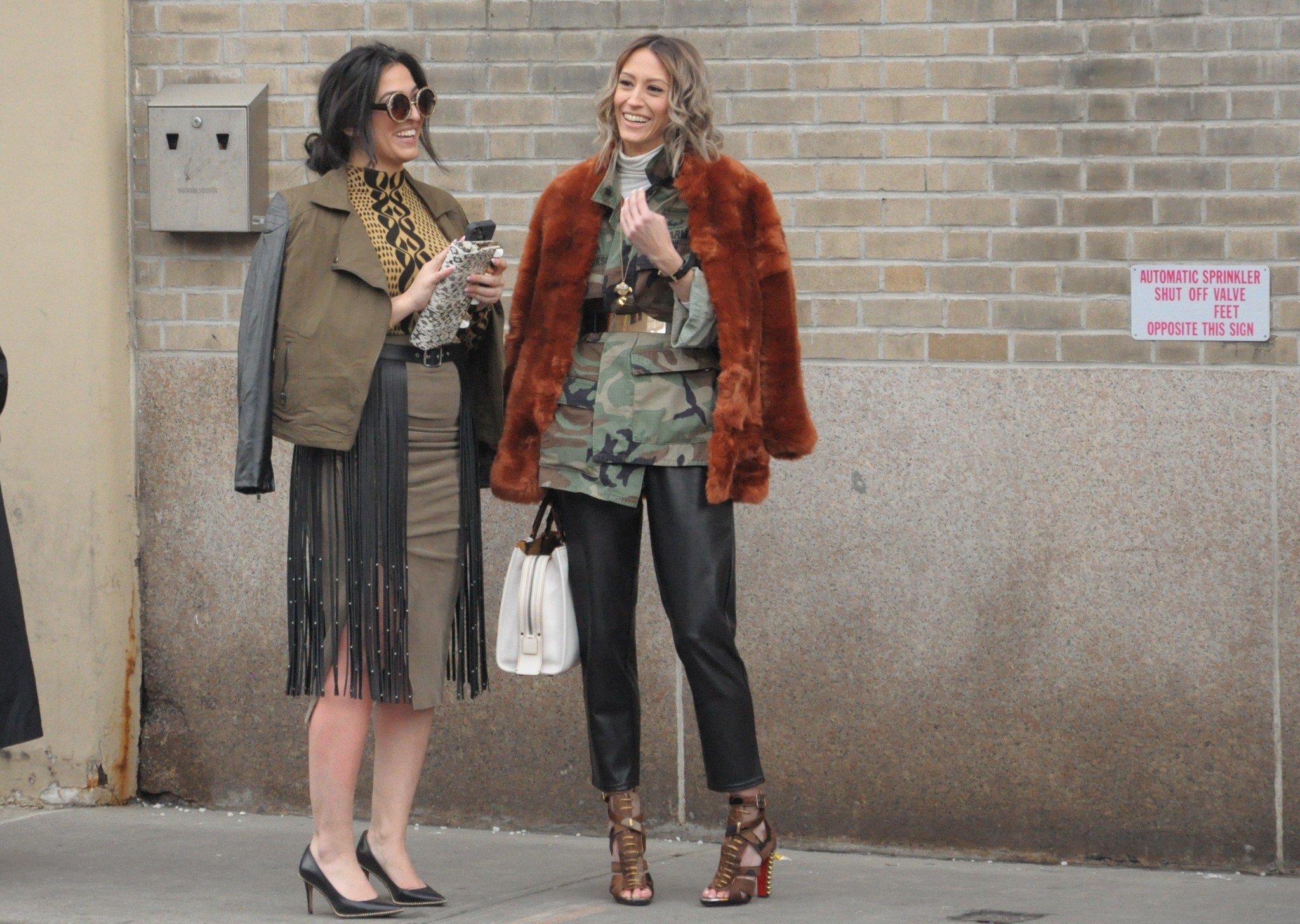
235;41;508;918
490;37;818;907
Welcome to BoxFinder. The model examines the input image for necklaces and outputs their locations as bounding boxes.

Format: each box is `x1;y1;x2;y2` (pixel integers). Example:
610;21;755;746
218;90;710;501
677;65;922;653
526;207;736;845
612;165;635;309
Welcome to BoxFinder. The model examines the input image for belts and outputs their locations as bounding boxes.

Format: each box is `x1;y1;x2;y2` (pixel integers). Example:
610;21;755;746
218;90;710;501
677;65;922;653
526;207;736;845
577;311;672;334
378;342;452;368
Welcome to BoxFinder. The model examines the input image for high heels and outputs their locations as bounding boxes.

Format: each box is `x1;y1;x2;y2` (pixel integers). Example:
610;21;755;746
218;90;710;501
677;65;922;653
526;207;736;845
700;788;777;906
355;829;447;907
601;787;654;905
300;844;403;918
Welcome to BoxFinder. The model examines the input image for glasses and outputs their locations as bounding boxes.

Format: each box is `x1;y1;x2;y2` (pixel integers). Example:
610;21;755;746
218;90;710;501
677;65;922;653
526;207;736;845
372;86;437;123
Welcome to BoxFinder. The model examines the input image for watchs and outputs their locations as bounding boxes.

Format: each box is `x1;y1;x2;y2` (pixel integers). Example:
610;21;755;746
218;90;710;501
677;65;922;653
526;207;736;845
658;251;697;283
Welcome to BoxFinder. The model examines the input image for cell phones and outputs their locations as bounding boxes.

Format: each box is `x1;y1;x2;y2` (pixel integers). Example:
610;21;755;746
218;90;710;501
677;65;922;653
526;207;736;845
464;220;496;241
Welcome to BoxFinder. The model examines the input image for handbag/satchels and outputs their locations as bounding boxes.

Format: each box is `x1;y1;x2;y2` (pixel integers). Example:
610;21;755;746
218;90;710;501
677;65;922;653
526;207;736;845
495;487;582;675
409;239;500;350
